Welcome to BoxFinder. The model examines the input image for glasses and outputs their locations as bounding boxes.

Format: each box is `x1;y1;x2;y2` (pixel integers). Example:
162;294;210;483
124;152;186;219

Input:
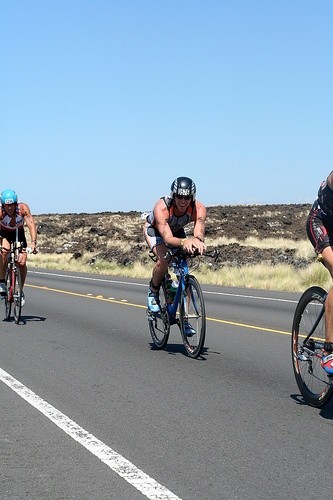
176;194;191;200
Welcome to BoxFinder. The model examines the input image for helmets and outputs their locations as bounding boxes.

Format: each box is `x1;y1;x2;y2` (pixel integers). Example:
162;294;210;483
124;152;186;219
0;190;17;204
171;177;196;201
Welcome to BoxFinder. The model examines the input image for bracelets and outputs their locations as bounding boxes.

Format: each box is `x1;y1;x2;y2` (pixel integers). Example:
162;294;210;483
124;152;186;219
180;238;187;246
31;240;37;245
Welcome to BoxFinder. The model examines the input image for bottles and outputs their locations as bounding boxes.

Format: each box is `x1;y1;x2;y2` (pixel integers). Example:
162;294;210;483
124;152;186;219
26;246;34;253
165;279;173;298
167;281;179;304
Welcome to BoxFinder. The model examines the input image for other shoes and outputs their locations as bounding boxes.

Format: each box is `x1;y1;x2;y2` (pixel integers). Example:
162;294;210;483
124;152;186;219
179;321;196;334
17;291;25;307
0;282;7;296
320;354;333;374
147;288;161;312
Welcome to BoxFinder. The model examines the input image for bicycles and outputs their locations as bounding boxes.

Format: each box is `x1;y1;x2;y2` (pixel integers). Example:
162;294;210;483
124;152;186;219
0;247;38;324
291;250;333;407
146;247;220;357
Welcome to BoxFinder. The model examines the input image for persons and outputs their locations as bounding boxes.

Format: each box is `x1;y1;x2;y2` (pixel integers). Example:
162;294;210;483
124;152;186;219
306;170;333;375
0;189;37;306
143;177;207;335
